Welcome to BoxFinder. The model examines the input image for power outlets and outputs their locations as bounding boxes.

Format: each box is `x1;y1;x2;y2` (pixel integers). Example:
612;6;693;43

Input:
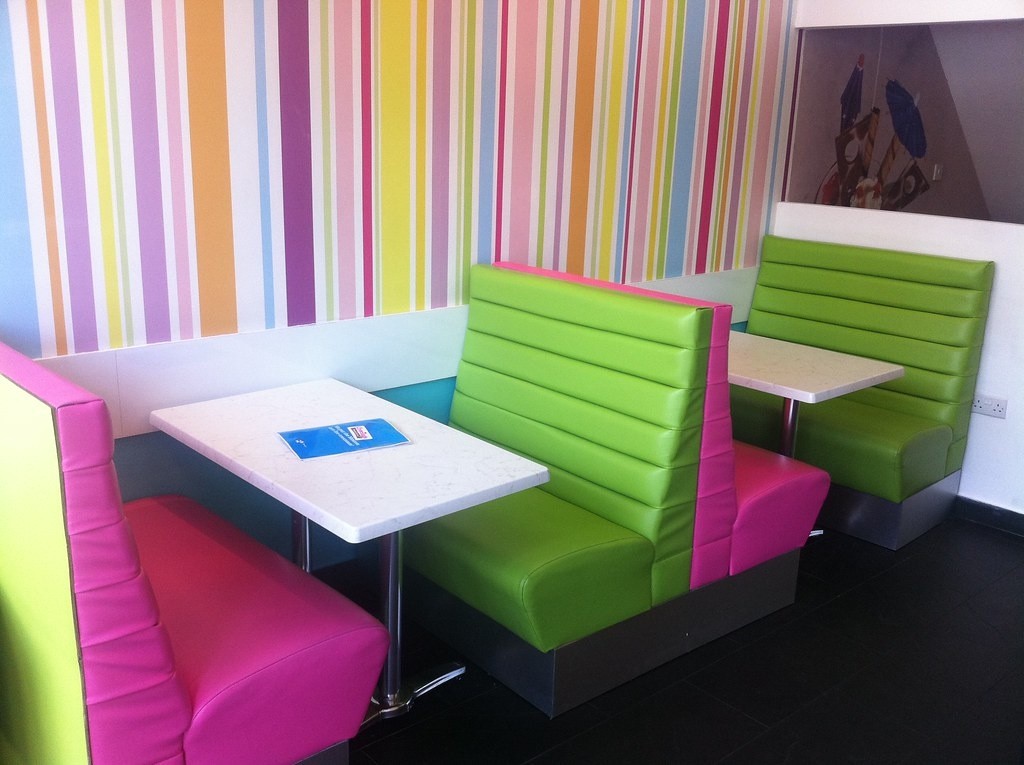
970;394;1008;419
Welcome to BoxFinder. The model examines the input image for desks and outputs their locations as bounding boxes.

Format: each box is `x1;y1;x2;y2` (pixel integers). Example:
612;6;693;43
151;378;550;733
727;329;903;540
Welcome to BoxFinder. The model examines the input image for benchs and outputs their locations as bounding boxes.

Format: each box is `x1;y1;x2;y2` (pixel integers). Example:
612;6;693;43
0;340;393;765
729;235;994;551
377;260;831;717
